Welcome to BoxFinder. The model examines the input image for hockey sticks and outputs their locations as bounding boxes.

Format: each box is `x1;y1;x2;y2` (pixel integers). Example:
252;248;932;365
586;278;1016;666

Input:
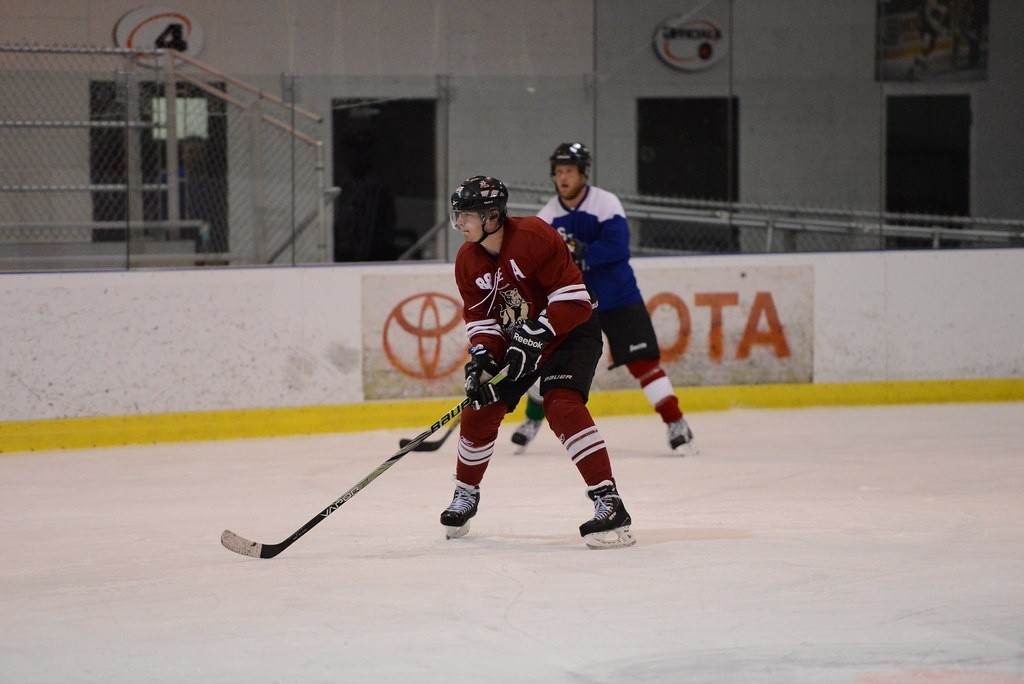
221;360;511;558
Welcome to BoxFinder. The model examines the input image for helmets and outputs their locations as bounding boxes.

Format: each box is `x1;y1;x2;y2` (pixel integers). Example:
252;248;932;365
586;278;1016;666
450;175;508;223
550;140;591;180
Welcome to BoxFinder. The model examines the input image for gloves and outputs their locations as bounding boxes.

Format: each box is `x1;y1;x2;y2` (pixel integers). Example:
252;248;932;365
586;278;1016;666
502;328;547;383
463;357;503;411
562;235;584;257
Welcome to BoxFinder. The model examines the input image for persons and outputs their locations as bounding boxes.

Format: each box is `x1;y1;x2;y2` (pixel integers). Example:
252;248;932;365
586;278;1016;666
510;141;694;451
948;1;987;72
906;1;953;80
440;175;631;538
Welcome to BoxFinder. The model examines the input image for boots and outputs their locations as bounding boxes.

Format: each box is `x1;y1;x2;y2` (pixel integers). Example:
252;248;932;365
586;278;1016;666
511;417;542;455
579;477;637;549
439;485;481;539
667;417;700;455
